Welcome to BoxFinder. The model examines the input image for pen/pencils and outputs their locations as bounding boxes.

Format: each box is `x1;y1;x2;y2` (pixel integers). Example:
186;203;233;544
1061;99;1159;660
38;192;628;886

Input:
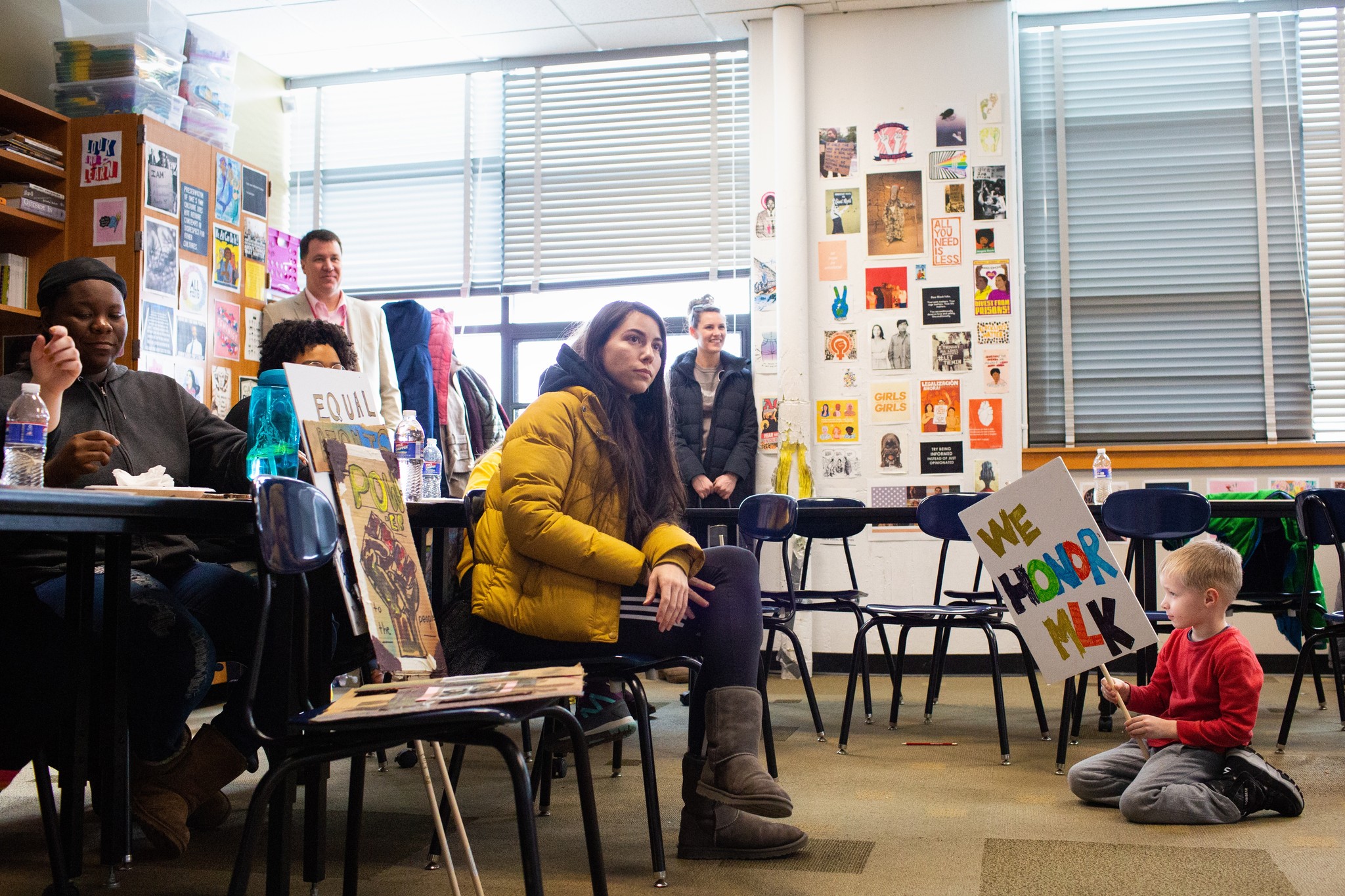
901;742;958;746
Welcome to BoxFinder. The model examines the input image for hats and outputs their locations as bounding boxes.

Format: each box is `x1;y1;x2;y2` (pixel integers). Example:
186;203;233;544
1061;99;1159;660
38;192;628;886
31;254;129;307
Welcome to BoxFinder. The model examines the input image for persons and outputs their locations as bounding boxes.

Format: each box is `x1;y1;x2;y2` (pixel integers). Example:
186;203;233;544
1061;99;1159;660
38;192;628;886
882;191;916;246
827;128;846;177
473;300;809;863
224;318;358;779
871;325;889;370
1066;541;1307;825
888;319;911;369
907;486;923;498
662;296;758;685
2;326;84;464
932;332;972;371
2;259;310;851
820;131;827;174
973;177;1006;219
261;230;404;684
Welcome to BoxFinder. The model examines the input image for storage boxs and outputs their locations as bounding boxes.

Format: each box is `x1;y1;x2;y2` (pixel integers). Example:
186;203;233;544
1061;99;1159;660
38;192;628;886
50;31;186;93
186;21;244;87
58;0;189;54
179;64;236;125
48;80;188;132
180;105;236;153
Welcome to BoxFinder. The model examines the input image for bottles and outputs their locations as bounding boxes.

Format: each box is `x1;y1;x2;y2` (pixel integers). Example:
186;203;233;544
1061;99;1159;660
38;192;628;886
246;369;301;496
1093;448;1113;505
394;410;425;503
423;438;443;498
1;383;49;489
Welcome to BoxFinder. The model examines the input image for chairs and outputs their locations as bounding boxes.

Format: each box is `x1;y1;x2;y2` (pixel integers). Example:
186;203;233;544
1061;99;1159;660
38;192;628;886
764;498;899;720
242;473;613;896
664;493;837;784
1047;488;1345;768
427;485;685;883
833;494;1054;767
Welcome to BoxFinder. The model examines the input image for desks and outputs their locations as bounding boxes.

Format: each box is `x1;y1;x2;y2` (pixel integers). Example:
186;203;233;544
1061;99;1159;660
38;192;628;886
673;499;920;534
1;484;474;896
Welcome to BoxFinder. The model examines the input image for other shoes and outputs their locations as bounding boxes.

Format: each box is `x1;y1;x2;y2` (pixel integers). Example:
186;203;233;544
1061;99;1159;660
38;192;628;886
616;688;657;719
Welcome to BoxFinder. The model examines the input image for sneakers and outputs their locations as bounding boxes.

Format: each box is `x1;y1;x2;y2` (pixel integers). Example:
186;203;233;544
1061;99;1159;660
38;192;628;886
1218;746;1304;820
554;693;635;754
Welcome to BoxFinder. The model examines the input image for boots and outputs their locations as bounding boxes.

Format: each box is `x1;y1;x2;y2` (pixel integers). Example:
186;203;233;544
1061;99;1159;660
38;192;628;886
140;725;233;834
673;746;811;864
696;681;792;818
129;722;250;861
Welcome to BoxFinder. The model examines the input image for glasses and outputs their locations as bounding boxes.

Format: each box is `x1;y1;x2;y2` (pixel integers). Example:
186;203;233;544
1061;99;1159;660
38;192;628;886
296;359;346;372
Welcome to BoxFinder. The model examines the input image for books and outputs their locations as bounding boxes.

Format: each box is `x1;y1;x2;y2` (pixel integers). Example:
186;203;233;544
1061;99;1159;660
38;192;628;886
1;129;67;309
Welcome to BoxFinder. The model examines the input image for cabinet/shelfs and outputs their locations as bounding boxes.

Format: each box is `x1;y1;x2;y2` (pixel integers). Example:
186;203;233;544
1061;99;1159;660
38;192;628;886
0;87;72;325
67;113;303;424
